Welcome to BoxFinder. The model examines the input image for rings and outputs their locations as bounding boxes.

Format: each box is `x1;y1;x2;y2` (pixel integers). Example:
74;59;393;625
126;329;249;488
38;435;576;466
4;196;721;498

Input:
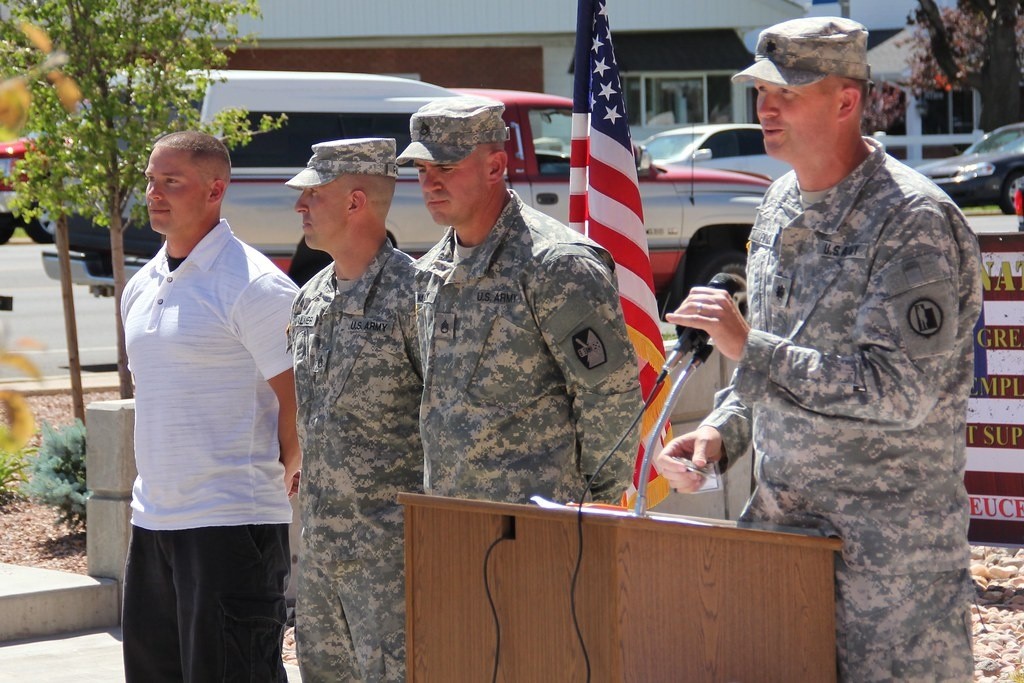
696;302;702;314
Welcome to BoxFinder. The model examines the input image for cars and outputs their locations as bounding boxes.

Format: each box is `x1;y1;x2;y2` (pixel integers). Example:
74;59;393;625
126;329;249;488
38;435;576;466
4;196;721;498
911;123;1024;216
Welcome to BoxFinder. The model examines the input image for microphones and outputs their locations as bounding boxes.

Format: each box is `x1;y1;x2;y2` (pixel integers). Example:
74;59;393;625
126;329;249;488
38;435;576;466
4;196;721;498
656;272;741;384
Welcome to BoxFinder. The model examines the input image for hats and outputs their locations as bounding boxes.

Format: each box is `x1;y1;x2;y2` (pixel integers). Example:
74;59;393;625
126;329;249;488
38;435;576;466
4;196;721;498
396;95;511;165
731;16;869;88
284;138;399;191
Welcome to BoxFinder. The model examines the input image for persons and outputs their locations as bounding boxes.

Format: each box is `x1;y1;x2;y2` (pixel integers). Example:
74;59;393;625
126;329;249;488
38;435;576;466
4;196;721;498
286;230;398;288
391;98;644;505
282;135;417;683
119;132;303;683
653;16;986;683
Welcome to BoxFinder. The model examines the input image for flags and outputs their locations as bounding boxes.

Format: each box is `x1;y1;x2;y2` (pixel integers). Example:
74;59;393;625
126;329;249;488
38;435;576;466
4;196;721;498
564;0;673;516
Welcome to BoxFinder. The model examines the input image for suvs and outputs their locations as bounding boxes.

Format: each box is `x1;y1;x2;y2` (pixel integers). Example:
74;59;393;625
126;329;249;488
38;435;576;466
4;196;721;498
0;67;794;364
